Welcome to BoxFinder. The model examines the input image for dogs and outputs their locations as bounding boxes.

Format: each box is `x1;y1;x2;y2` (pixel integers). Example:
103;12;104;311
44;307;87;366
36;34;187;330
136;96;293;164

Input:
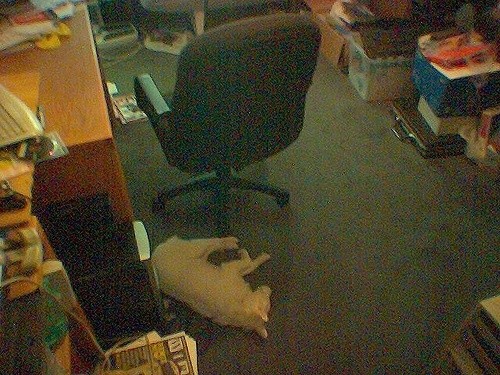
150;235;273;339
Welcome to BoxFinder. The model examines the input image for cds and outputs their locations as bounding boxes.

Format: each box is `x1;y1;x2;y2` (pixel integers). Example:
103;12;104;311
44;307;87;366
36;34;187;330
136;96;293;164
37;135;59;161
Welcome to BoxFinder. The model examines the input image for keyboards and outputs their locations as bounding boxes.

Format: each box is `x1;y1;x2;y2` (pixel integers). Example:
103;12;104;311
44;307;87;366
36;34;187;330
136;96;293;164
0;84;44;148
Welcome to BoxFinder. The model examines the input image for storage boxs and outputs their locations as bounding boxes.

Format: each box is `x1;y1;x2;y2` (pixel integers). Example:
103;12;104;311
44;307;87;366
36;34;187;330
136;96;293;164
410;46;500;117
305;0;411;74
348;37;419;101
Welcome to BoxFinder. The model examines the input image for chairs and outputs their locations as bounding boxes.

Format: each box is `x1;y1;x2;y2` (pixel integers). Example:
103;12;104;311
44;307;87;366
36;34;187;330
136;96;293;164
135;12;322;238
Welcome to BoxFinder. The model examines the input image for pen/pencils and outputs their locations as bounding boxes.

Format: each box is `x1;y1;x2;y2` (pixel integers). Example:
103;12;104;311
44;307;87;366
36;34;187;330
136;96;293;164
37;106;45;130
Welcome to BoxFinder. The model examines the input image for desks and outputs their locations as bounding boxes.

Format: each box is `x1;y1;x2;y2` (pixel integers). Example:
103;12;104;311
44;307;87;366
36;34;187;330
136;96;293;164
0;0;134;224
